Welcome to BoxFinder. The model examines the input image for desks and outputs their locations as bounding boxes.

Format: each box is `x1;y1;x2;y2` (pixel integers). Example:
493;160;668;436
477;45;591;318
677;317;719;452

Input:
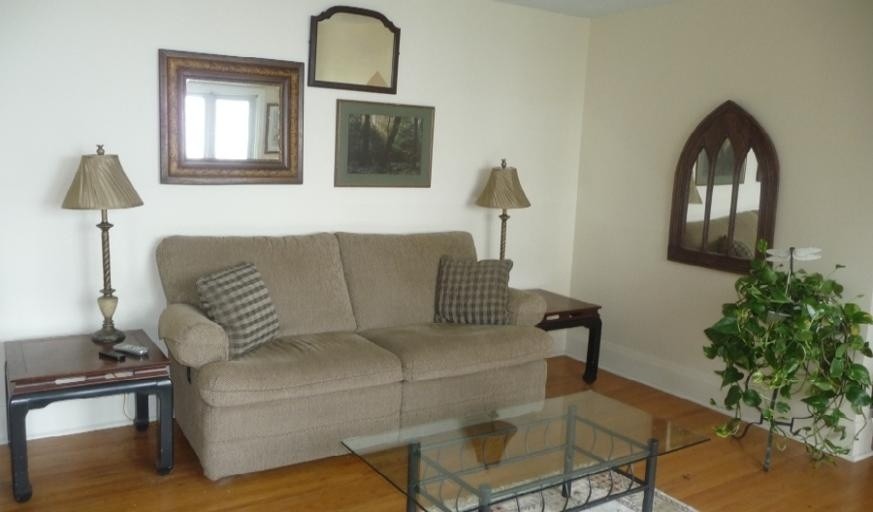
3;328;174;503
519;288;602;384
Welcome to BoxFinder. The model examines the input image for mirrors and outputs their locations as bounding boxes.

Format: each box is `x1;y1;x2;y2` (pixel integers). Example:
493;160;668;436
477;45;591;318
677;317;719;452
667;97;779;274
158;49;304;184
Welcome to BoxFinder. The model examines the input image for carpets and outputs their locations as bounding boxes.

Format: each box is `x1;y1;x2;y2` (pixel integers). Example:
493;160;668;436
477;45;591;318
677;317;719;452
414;456;698;512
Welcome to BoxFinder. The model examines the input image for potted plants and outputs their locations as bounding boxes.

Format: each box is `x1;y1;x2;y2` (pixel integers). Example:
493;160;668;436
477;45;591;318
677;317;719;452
703;238;872;473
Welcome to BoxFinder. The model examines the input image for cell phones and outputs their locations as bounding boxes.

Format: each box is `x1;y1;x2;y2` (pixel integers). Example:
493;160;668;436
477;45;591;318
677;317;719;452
98;350;126;361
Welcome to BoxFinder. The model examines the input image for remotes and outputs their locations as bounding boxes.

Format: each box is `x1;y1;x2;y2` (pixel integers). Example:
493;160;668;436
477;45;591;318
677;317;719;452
112;341;148;357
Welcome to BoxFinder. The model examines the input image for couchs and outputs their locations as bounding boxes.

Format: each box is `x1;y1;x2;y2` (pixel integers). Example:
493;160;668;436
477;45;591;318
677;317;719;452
155;230;554;483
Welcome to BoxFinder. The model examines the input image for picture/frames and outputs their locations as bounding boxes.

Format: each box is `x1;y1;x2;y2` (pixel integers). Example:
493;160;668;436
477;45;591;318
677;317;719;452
329;99;436;189
263;103;280;154
307;5;400;95
695;138;747;186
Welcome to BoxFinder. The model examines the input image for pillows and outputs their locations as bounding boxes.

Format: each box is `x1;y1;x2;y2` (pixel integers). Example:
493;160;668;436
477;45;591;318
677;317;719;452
434;252;514;326
194;260;280;359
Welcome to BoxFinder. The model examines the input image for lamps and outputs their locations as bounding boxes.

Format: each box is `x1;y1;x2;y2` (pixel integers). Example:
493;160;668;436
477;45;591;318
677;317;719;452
61;144;143;345
475;158;532;260
460;419;518;470
